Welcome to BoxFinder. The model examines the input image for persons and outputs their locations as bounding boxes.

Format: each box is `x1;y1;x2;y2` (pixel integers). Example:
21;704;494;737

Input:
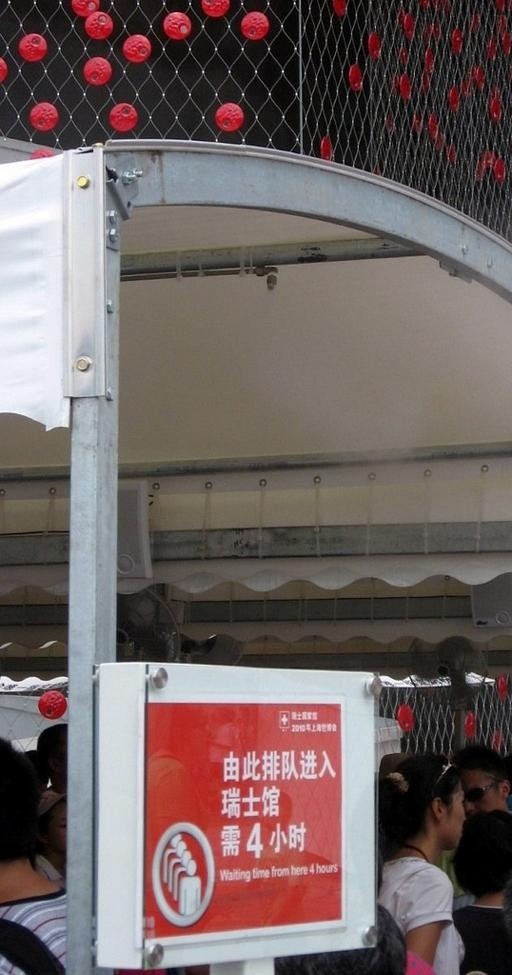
274;744;511;973
0;724;68;974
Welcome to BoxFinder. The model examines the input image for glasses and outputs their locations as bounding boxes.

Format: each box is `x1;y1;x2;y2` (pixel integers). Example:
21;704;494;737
466;779;497;803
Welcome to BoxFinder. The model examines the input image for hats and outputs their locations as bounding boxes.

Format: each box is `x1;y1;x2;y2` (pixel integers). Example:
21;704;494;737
36;791;67;817
